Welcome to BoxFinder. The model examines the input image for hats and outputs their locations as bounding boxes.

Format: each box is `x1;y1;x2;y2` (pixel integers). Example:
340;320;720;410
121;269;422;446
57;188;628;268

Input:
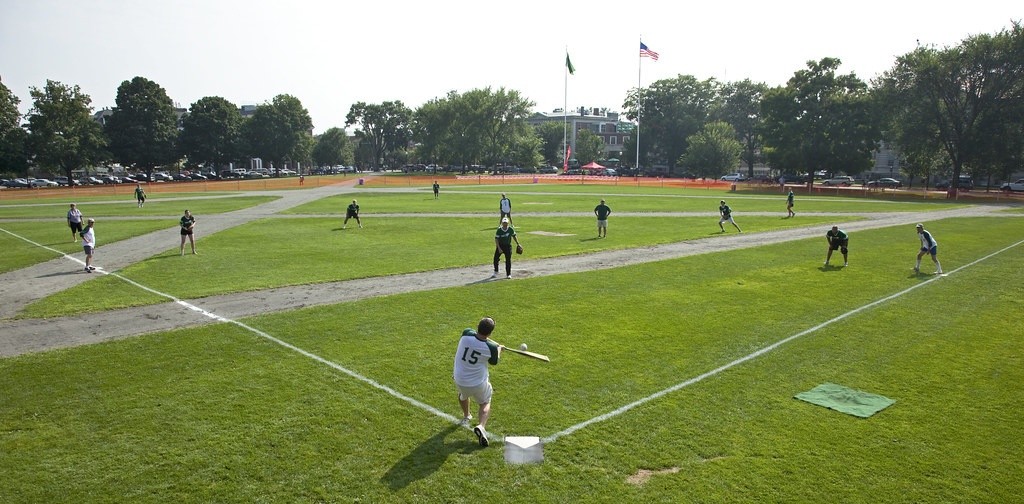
917;223;924;228
502;217;509;223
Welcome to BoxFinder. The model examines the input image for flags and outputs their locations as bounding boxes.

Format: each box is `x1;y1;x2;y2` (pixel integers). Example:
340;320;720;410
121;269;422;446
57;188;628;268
566;52;577;76
640;41;659;62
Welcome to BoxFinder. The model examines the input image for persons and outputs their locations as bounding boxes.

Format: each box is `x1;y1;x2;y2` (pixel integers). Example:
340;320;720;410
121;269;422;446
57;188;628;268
79;218;95;273
500;193;514;228
180;210;198;256
594;199;612;238
454;318;505;446
433;180;440;199
492;218;523;279
824;225;849;266
701;175;706;185
785;187;795;217
748;172;785;193
946;181;953;200
910;222;942;273
861;179;878;191
299;174;304;186
718;200;742;233
342;199;363;229
134;184;147;208
67;203;84;242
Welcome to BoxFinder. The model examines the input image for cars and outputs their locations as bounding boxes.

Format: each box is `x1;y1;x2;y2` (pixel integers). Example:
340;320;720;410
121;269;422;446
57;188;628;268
0;165;357;188
539;166;558;174
383;165;388;170
746;174;772;184
936;175;974;192
401;164;415;173
774;169;808;184
488;163;503;173
602;166;640;178
506;166;517;172
819;170;827;178
426;164;443;173
414;164;426;172
868;178;901;189
1000;179;1024;192
517;165;537;173
721;173;745;182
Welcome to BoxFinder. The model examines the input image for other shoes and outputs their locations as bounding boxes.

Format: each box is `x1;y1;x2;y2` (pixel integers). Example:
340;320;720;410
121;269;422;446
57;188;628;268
507;275;511;278
492;272;499;278
181;250;185;256
192;250;198;255
462;414;472;424
89;266;95;270
823;261;829;265
910;266;920;271
844;262;848;266
474;425;489;447
84;267;92;273
934;270;942;274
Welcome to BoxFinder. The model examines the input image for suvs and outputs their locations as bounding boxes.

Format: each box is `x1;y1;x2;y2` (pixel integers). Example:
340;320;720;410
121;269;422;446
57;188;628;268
823;176;854;188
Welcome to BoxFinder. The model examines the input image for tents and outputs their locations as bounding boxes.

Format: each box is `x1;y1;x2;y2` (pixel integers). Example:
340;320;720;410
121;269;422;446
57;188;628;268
581;160;606;184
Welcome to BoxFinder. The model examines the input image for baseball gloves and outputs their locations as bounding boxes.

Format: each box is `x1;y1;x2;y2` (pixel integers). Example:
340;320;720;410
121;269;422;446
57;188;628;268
841;247;848;254
188;225;193;231
516;245;523;254
144;195;147;198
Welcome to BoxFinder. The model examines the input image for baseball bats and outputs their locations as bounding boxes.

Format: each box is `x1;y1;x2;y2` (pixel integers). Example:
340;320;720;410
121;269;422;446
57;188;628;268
504;346;550;362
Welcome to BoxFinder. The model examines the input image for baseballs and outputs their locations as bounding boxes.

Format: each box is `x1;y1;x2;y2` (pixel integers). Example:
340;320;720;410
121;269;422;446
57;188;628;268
521;343;527;351
